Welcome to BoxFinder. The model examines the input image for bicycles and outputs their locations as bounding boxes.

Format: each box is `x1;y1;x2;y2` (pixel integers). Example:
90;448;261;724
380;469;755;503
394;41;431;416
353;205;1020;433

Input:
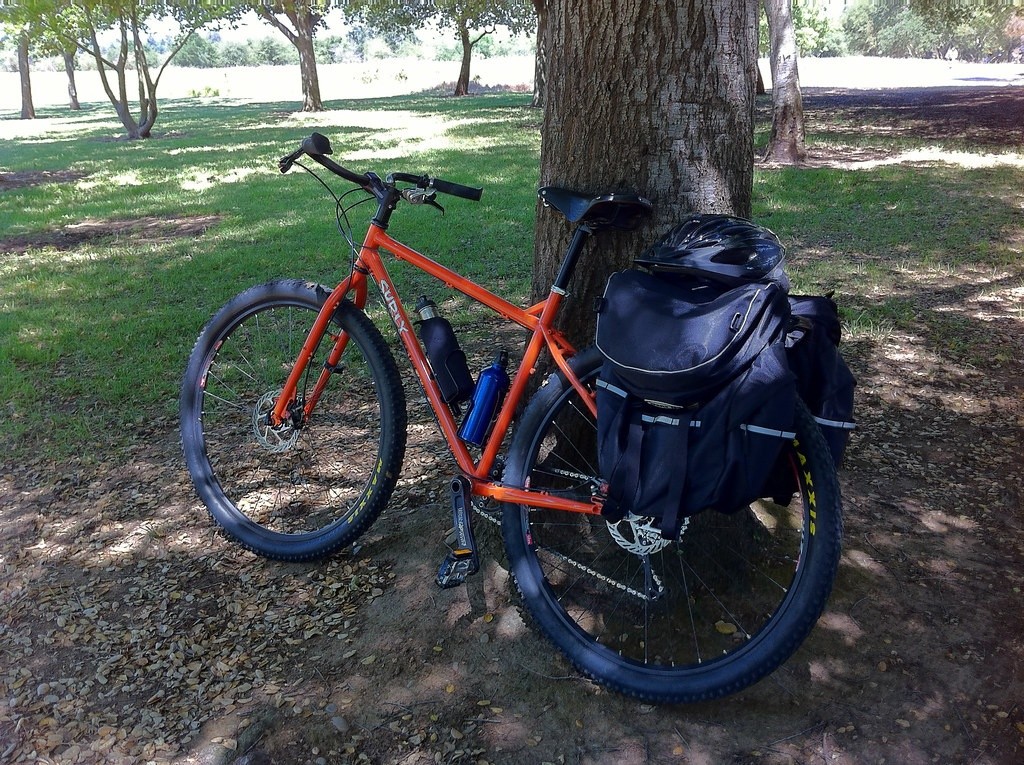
179;132;846;706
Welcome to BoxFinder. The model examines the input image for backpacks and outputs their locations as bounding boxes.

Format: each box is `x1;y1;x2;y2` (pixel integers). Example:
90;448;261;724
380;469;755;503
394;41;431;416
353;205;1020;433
594;214;790;410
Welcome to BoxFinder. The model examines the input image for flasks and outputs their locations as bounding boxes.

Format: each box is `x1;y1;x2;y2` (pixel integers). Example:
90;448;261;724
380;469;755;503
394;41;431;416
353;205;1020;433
413;293;476;406
456;347;513;448
